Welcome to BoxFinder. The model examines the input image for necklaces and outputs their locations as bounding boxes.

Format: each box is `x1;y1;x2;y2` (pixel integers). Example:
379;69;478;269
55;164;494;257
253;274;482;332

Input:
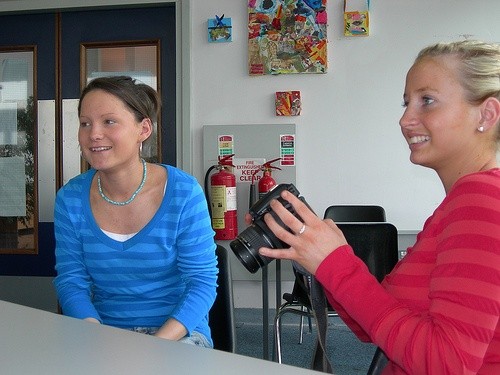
98;158;147;205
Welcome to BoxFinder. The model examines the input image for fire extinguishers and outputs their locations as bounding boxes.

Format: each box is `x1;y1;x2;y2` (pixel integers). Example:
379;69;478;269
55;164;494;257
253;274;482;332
210;153;238;241
258;157;282;201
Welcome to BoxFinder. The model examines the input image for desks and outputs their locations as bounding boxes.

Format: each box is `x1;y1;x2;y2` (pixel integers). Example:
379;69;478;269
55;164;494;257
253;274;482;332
0;300;333;375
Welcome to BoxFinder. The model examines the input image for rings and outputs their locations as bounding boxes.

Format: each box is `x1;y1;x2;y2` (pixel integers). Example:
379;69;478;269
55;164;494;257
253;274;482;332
296;224;305;235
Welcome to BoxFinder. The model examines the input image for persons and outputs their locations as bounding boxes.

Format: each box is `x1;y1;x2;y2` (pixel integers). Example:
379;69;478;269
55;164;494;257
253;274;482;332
245;40;500;375
53;75;219;349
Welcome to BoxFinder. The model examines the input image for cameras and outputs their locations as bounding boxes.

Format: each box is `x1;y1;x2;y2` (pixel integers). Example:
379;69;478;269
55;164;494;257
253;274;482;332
230;184;317;274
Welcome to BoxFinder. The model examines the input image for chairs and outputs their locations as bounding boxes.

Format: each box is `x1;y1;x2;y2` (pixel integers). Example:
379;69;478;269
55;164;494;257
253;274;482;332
275;205;399;364
208;243;237;353
